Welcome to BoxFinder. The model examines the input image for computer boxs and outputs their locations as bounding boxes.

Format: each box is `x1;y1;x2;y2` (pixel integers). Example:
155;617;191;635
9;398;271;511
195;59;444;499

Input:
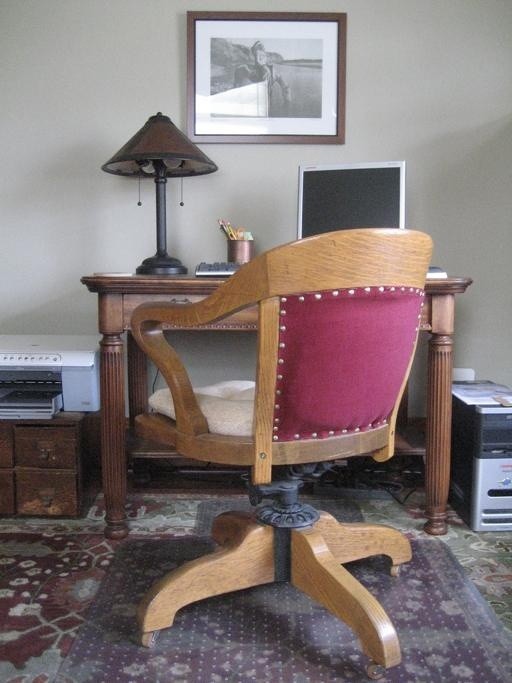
453;379;512;533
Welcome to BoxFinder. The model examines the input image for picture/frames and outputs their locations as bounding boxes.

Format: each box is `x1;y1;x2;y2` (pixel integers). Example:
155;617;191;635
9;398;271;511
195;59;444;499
188;10;347;144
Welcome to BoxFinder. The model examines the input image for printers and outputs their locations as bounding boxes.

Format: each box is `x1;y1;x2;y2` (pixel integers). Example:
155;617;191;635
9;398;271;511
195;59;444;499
1;334;99;421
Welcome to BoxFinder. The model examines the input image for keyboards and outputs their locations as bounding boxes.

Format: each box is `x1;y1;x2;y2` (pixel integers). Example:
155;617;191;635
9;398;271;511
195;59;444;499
193;262;251;276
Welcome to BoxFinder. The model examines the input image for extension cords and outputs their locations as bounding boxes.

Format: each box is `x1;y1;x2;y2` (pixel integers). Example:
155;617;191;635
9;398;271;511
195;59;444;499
279;470;337;485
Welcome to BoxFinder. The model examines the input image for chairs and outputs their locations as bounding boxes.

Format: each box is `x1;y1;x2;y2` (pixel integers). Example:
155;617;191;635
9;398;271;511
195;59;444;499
131;228;434;669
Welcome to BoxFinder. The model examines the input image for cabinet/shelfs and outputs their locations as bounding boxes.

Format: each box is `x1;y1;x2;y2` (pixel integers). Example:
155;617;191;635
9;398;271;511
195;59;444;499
0;412;89;517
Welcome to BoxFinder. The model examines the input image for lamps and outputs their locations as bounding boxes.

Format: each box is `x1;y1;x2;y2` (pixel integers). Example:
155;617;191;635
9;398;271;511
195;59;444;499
101;111;218;276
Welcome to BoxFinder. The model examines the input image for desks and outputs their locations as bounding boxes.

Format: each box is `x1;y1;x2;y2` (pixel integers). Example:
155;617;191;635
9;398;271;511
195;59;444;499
81;273;473;540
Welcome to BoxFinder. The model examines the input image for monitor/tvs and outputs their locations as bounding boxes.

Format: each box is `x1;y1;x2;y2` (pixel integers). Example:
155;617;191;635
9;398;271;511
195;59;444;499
297;159;405;243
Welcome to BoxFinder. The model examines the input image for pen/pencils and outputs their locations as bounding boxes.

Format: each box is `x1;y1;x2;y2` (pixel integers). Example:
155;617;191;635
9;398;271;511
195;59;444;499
218;218;246;240
